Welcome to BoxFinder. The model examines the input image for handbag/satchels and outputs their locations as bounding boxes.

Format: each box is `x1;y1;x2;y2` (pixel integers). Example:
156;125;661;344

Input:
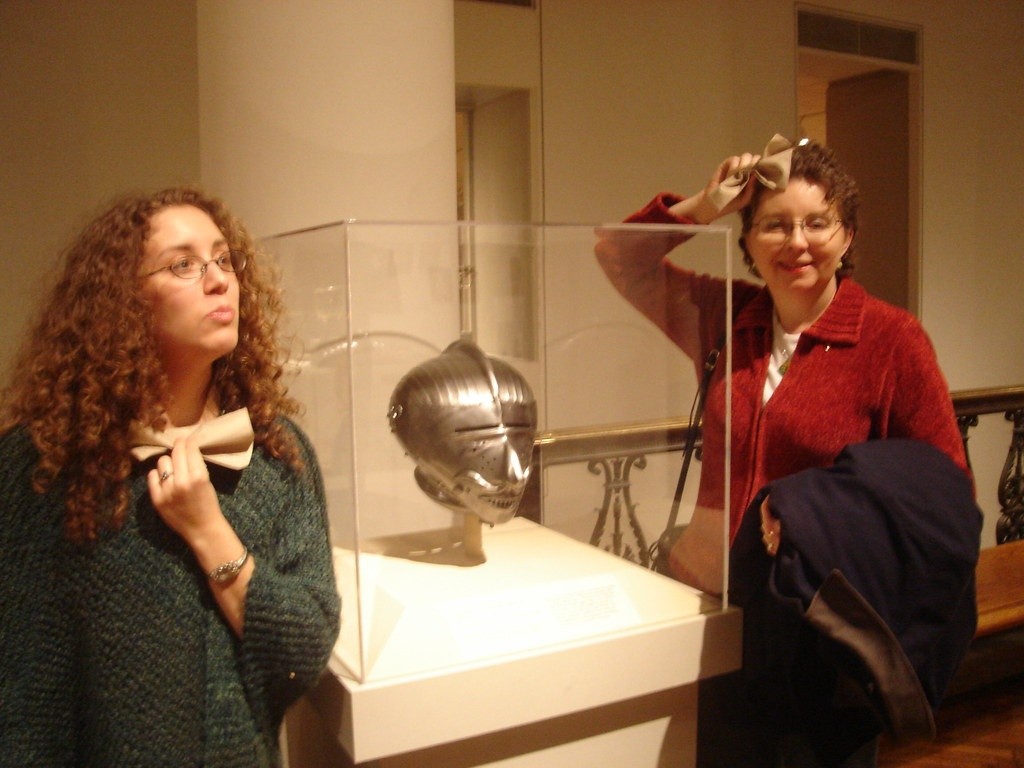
648;524;692;572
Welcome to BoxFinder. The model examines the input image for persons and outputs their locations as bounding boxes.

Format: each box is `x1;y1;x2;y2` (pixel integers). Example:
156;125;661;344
388;338;538;524
2;188;342;768
596;138;975;768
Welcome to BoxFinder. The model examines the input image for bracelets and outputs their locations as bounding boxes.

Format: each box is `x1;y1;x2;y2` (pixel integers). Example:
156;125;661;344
207;545;248;583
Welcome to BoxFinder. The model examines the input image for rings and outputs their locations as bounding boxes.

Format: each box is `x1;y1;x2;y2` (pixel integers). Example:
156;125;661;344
769;531;773;536
767;543;773;551
160;471;174;481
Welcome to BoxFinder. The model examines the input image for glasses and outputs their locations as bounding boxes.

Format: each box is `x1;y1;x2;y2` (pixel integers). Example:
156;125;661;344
748;211;848;240
136;249;249;281
